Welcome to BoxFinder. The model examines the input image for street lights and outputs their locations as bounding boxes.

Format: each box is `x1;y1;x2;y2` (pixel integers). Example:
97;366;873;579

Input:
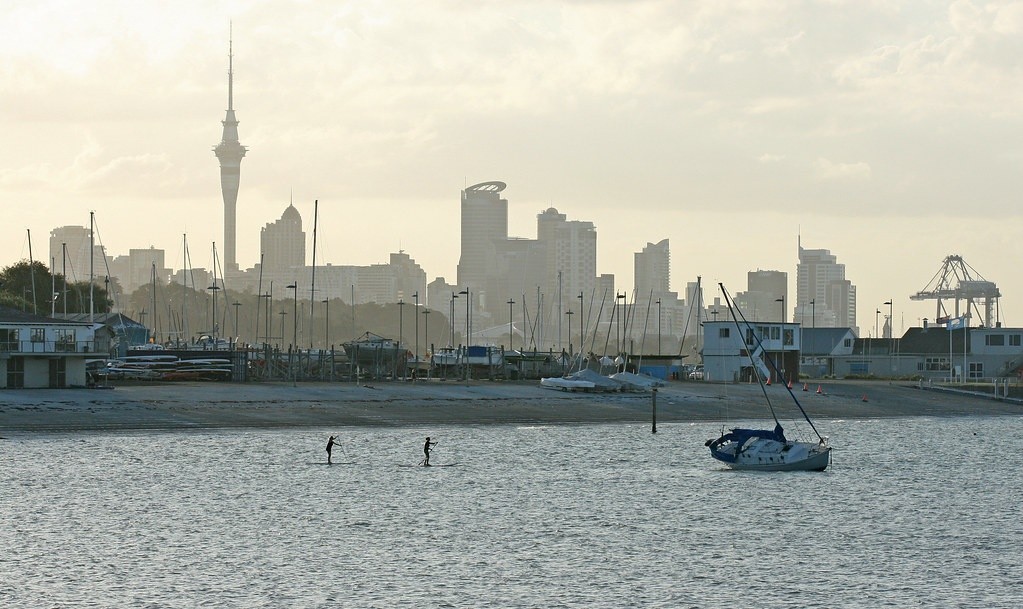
616;290;626;352
206;281;220;339
774;294;785;383
262;292;272;343
507;298;515;349
875;307;881;344
423;308;431;354
566;311;574;355
449;286;469;351
884;299;892;354
232;300;243;337
321;296;329;349
656;297;661;354
577;290;584;357
412;291;419;372
286;280;297;351
279;309;287;347
105;273;109;351
810;298;814;364
396;299;405;348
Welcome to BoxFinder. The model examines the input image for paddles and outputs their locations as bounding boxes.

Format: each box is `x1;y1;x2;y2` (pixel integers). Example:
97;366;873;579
338;436;348;461
417;442;438;466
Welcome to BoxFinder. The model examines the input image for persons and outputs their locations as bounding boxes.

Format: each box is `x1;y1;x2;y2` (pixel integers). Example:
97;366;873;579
325;436;342;463
424;437;438;466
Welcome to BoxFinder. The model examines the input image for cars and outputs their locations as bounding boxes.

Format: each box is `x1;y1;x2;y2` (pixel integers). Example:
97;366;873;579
682;363;704;379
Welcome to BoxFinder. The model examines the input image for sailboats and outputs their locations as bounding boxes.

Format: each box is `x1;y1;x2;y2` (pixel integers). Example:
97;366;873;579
541;283;671;393
84;200;504;380
704;282;831;471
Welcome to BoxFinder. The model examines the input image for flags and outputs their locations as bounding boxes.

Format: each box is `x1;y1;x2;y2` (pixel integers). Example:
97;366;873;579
937;316;949;325
947;316;964;331
